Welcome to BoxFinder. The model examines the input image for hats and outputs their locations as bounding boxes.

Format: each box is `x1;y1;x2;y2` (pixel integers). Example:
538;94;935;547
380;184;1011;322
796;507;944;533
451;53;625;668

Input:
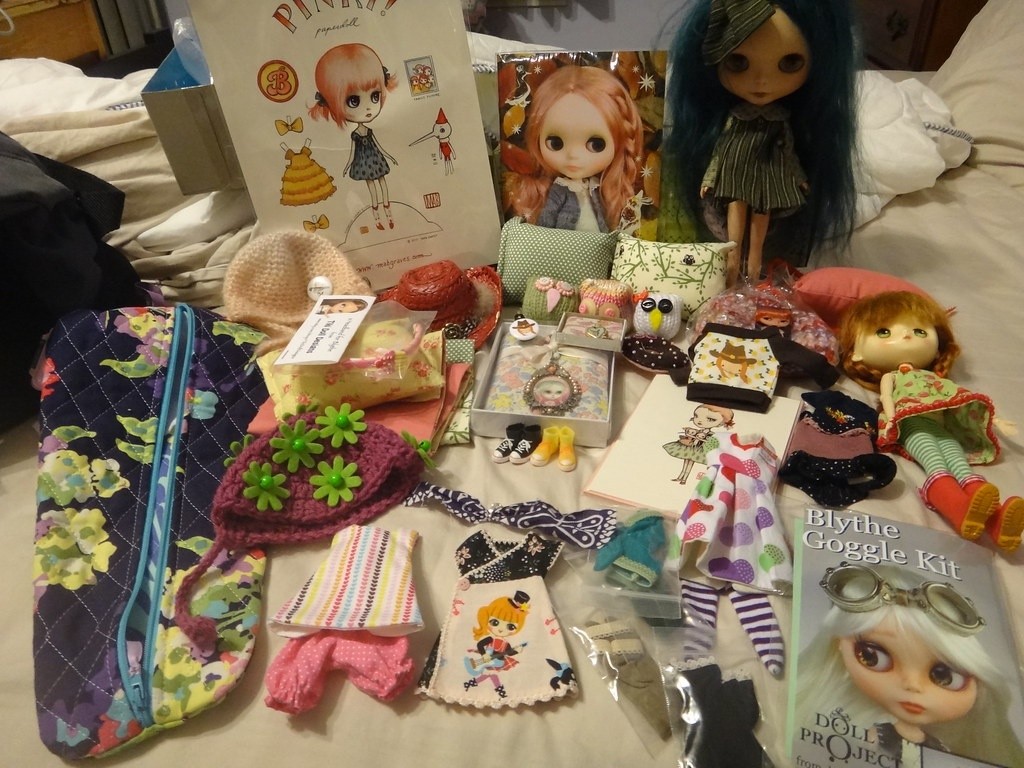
375;261;502;351
176;400;436;649
222;230;376;359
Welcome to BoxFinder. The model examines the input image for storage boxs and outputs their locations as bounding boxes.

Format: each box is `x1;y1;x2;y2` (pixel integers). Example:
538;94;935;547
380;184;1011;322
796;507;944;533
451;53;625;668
555;312;627;353
470;320;616;449
140;37;247;196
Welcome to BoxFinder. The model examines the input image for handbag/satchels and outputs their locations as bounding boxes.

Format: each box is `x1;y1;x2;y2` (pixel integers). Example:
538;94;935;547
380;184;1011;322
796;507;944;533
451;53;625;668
33;299;266;759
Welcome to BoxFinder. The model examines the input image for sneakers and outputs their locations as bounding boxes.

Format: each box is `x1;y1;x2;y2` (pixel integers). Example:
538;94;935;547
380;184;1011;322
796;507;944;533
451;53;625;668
492;423;525;463
509;424;542;464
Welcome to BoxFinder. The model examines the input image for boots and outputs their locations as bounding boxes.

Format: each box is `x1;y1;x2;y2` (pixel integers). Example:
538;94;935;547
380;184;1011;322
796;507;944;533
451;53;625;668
559;426;576;471
958;474;1024;553
531;426;562;467
920;470;1000;541
